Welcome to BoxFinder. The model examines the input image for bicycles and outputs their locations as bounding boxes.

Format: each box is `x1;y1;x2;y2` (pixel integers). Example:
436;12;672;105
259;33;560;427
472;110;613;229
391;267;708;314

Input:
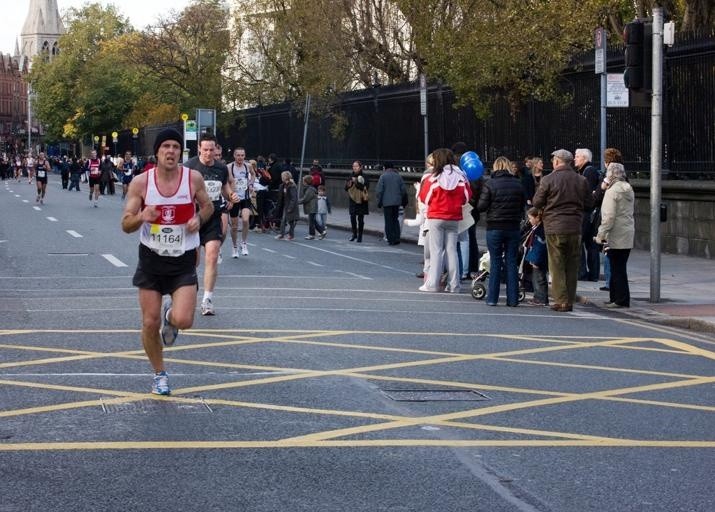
222;181;294;238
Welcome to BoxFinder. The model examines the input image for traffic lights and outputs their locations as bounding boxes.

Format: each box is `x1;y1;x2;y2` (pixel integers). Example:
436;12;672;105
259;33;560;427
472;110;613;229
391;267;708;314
621;18;652;94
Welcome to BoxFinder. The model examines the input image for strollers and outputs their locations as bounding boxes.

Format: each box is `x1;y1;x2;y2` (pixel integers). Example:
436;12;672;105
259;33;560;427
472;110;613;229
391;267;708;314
468;217;533;304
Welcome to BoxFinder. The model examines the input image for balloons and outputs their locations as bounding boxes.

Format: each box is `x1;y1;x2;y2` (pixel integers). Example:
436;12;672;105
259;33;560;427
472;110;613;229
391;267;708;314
461;159;484;181
459;151;480;171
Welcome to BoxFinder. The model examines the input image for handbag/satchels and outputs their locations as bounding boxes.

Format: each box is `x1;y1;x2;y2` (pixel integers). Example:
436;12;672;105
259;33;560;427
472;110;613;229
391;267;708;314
457;203;475;233
348;184;369;204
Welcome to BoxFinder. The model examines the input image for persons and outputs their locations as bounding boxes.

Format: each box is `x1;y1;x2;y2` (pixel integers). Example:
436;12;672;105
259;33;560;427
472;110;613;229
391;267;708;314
500;156;552;293
226;147;256;259
592;148;628;291
532;149;595;311
375;161;408;245
309;159;331;238
296;175;328;240
476;156;527;307
0;147;156;209
593;162;634;310
403;148;486;293
221;153;300;241
178;133;240;315
213;144;235;265
121;127;215;396
343;160;370;243
574;148;602;282
525;207;550;306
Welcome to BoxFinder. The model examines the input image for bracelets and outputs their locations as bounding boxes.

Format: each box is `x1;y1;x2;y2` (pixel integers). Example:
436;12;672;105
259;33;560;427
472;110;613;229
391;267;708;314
195;212;203;228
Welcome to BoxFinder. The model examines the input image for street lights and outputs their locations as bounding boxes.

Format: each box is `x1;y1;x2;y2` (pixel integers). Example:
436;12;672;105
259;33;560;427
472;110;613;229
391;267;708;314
27;90;32;150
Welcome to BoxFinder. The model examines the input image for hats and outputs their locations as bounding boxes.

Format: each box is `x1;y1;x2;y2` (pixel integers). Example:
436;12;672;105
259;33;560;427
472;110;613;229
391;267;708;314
153;128;184;159
551;149;573;161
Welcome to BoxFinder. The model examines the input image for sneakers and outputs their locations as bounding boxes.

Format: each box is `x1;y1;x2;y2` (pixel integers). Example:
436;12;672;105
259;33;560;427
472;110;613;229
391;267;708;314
36;196;44;204
241;243;247;255
416;272;486;293
217;251;223;263
275;225;327;240
201;298;216;316
89;193;98;207
349;236;361;241
152;370;171;395
160;299;178;345
600;286;618;308
486;298;573;312
232;246;240;257
